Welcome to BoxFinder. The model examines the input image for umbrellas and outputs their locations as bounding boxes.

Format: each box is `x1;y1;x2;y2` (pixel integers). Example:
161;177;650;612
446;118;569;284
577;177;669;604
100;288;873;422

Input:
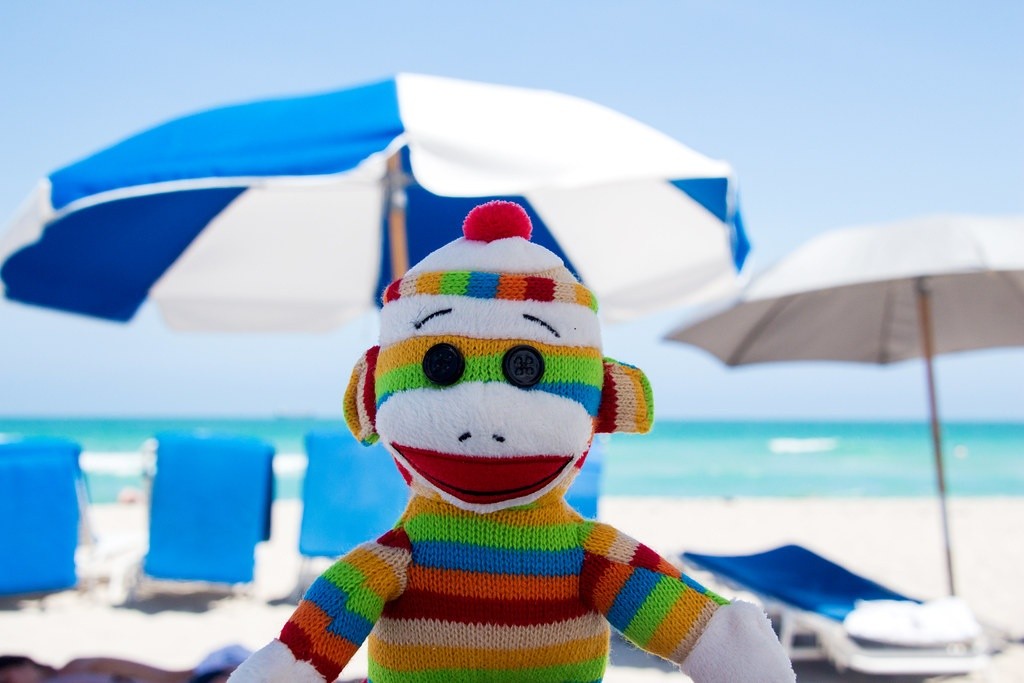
1;71;750;347
661;215;1023;599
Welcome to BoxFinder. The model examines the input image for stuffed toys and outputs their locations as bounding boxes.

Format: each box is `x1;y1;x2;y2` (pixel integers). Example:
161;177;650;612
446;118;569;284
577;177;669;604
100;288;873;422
223;201;799;683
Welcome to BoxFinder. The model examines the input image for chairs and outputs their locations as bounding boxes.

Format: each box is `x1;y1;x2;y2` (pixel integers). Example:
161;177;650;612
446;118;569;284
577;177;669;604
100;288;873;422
0;436;108;599
126;434;278;605
679;544;987;677
287;429;409;603
